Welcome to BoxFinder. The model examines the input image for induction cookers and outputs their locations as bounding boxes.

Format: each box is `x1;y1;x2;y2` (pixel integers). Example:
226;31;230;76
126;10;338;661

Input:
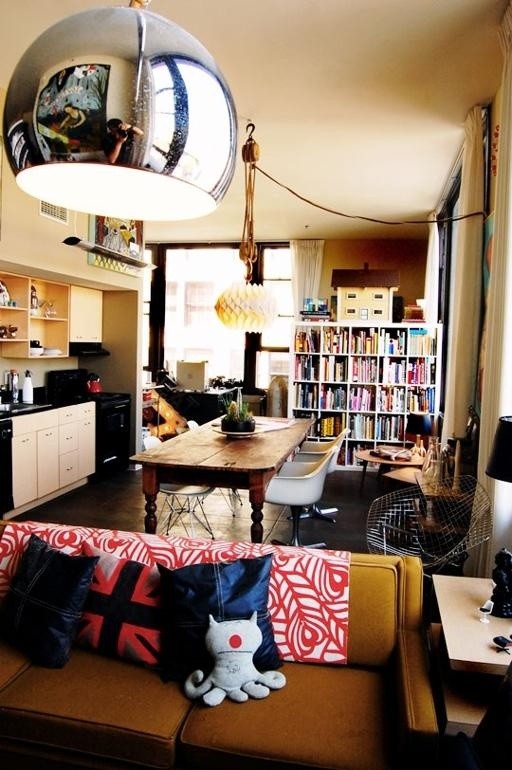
50;392;132;410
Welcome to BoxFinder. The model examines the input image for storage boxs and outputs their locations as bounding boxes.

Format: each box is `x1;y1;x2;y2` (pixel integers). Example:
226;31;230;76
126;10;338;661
176;357;210;390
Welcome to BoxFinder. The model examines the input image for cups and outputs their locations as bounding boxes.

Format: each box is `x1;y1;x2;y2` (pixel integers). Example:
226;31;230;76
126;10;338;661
317;298;328;312
308;303;315;311
31;340;40;348
303;299;313;311
7;373;20;403
144;392;152;401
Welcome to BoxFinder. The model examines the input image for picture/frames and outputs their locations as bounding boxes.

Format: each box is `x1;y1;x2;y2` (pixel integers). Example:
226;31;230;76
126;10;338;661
87;212;149;280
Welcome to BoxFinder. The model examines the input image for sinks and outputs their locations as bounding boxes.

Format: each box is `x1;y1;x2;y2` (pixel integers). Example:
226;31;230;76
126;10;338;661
0;403;53;414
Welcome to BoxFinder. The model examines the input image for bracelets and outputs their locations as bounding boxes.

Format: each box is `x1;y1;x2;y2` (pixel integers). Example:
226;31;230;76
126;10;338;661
129;124;134;129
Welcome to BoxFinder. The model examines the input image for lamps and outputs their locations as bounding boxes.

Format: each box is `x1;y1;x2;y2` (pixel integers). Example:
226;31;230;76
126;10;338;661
484;414;512;619
405;410;433;459
59;209;159;277
2;1;243;226
212;120;290;334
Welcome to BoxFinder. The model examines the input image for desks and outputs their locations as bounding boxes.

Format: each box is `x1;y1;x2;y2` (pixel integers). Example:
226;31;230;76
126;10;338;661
127;411;319;544
154;384;239;426
353;447;428;493
430;571;512;741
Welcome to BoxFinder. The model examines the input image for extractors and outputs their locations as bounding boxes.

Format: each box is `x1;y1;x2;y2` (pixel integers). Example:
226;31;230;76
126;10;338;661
69;342;110;356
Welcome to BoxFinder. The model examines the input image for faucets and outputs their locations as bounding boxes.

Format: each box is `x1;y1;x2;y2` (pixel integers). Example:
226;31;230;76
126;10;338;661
0;384;6;404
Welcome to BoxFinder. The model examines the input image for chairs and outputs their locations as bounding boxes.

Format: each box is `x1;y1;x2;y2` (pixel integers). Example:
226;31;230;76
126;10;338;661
287;428;354;524
187;416;245;516
263;445;339;550
143;433;217;542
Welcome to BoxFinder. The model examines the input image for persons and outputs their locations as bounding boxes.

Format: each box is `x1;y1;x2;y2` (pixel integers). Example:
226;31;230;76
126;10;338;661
102;118;144;166
59;103;92;141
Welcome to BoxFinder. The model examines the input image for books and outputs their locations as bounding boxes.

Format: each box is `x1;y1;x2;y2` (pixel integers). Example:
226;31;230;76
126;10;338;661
291;298;436;466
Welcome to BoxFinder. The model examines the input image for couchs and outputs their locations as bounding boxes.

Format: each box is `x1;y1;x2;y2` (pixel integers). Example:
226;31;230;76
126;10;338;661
1;519;440;767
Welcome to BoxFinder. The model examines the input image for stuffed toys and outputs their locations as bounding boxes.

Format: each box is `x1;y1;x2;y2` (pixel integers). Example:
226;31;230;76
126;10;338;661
186;608;286;707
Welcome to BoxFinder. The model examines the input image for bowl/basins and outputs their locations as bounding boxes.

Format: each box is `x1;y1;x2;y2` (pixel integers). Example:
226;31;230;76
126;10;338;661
31;348;44;356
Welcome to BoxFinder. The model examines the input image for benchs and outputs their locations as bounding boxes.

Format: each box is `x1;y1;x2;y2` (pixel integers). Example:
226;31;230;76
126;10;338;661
382;467;424;488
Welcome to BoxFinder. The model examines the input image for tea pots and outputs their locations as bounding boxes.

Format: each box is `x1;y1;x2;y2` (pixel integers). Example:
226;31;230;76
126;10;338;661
85;373;103;396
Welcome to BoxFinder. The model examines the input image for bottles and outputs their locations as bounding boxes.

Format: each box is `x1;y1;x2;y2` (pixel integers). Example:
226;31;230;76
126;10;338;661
45;306;58;318
410;435;455;494
142;427;151;442
31;291;39;308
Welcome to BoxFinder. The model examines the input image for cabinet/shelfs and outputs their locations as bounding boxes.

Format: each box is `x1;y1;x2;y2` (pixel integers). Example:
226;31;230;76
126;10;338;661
8;401;96;518
0;271;70;359
413;471;469;538
289;321;443;473
70;283;103;344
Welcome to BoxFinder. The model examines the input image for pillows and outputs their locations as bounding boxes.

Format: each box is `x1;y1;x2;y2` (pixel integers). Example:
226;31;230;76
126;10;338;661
152;551;292;685
69;540;166;672
2;530;104;671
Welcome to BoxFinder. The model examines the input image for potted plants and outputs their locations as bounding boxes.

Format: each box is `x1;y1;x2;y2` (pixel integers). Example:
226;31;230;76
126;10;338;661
216;390;256;432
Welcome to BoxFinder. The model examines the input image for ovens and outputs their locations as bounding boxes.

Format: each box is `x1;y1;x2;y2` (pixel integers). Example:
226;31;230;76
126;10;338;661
97;400;131;474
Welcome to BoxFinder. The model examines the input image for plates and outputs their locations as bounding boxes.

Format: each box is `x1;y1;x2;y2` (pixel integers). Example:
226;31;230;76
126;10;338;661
45;349;62;355
1;280;11;306
214;427;265;439
